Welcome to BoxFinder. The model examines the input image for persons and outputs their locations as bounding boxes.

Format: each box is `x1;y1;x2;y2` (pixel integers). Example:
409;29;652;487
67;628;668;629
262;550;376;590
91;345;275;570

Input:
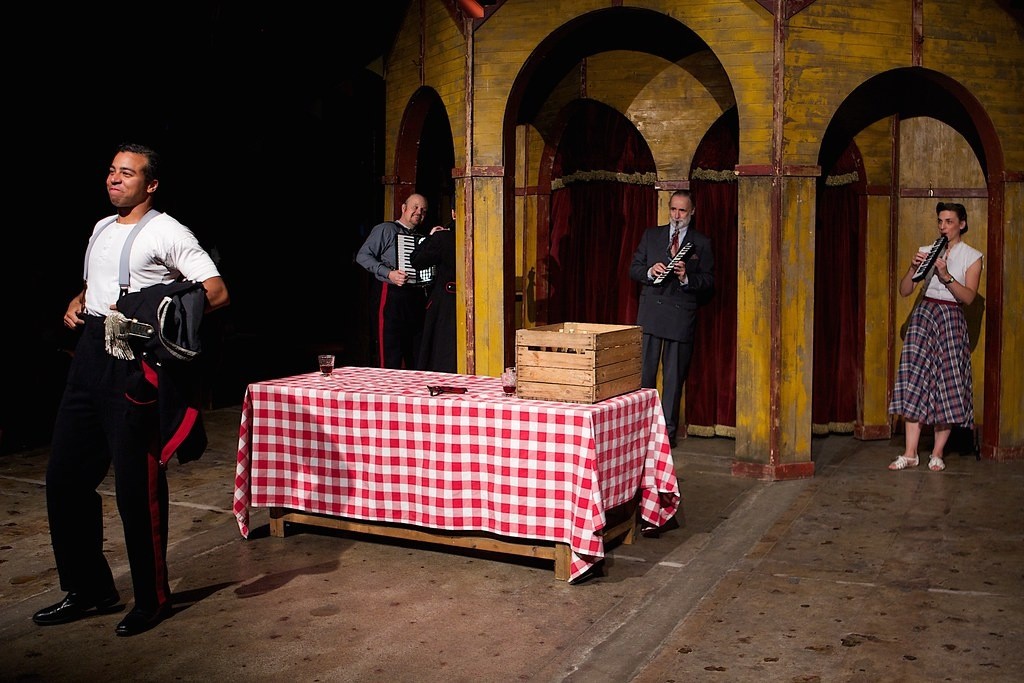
887;201;985;471
356;192;457;374
629;189;715;449
32;143;232;637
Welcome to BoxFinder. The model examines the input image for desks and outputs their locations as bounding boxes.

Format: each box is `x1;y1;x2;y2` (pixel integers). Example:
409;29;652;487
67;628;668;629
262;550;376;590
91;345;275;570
246;366;660;581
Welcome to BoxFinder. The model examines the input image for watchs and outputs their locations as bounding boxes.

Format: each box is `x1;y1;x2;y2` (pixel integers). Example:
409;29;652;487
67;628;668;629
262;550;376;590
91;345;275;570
943;276;955;285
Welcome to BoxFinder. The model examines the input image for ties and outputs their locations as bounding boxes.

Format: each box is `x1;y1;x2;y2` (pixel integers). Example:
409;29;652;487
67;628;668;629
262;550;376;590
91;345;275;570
670;229;679;258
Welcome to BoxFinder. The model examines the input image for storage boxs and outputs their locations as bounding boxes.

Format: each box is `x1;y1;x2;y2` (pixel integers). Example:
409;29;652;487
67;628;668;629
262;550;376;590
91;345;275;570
514;322;644;404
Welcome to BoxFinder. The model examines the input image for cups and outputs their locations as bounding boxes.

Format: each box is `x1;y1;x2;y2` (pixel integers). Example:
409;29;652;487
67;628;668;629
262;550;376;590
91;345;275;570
506;367;516;375
501;373;516;397
318;355;335;375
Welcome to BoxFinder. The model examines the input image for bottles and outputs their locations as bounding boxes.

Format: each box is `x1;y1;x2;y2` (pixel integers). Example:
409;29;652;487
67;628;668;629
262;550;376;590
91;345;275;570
580;331;587;354
546;329;555;351
557;329;565;353
567;329;578;354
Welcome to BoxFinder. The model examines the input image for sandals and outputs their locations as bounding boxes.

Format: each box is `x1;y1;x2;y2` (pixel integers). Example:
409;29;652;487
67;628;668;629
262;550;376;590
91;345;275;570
928;454;945;471
888;454;919;471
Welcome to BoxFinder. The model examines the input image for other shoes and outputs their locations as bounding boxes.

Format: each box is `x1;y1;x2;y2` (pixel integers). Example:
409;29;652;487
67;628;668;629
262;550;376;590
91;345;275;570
670;437;676;449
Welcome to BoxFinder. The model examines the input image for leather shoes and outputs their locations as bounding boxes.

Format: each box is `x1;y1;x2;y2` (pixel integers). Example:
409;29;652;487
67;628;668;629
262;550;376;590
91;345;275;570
116;603;172;635
32;591;120;624
